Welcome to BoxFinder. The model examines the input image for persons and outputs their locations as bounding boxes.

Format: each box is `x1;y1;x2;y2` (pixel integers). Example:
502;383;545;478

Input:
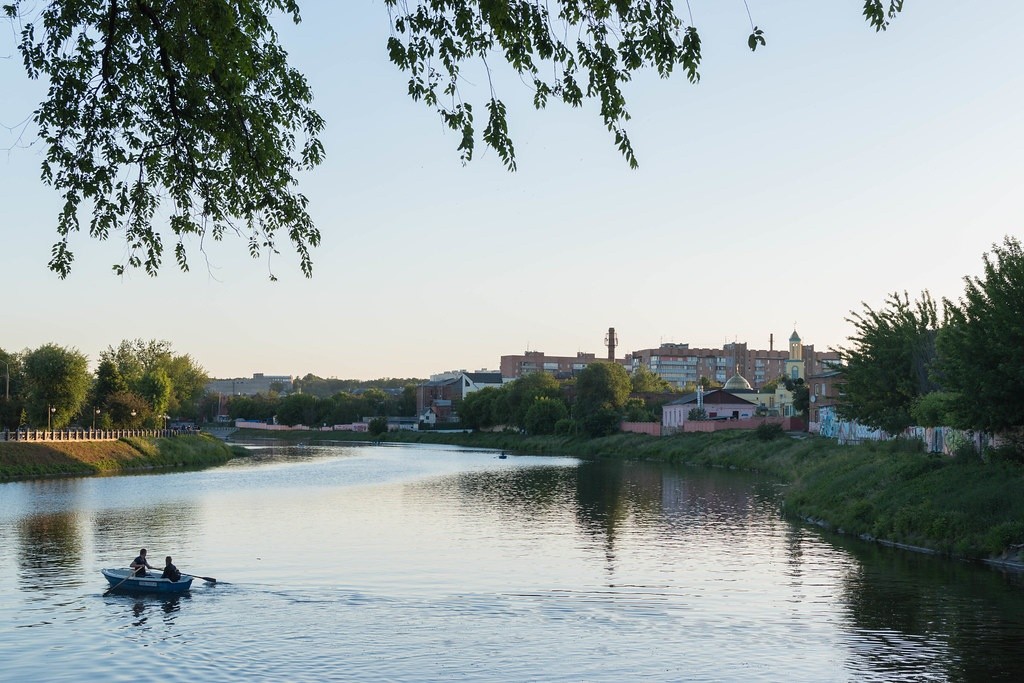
130;549;154;578
161;556;180;582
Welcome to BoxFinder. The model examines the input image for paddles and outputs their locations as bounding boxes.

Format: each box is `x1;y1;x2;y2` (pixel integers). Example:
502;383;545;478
102;563;143;599
148;564;216;583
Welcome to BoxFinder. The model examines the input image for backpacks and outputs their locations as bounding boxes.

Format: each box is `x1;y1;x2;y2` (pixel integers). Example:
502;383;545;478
175;570;181;580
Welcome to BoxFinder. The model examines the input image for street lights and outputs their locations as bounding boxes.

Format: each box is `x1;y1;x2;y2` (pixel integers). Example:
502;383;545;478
165;414;171;431
47;404;56;432
93;405;100;431
131;408;136;429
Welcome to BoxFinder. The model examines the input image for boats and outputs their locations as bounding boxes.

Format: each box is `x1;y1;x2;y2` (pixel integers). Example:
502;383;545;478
100;566;195;594
499;456;507;459
